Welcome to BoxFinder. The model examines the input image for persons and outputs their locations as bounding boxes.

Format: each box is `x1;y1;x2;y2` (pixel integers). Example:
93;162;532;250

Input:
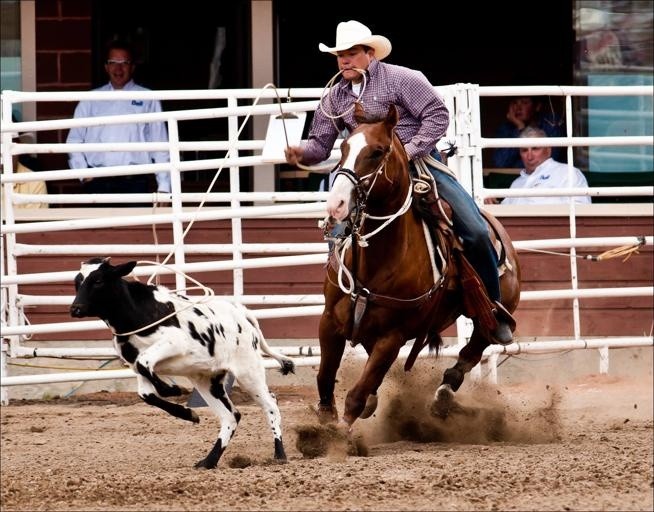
0;127;50;211
64;41;173;207
484;126;592;206
283;21;515;346
484;96;564;188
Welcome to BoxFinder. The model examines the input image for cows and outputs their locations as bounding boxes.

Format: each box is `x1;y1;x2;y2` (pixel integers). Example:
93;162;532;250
69;255;296;471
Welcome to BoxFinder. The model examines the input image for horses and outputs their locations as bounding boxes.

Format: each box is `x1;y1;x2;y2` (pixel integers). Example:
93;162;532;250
316;102;522;436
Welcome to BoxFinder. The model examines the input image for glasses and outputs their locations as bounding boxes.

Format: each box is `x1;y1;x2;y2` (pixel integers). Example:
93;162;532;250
105;59;132;66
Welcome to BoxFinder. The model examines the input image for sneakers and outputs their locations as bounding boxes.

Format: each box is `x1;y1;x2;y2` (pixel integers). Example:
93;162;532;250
483;307;516;345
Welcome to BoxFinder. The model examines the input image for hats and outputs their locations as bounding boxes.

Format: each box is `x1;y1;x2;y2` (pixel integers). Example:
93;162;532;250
0;122;33;140
314;20;392;65
